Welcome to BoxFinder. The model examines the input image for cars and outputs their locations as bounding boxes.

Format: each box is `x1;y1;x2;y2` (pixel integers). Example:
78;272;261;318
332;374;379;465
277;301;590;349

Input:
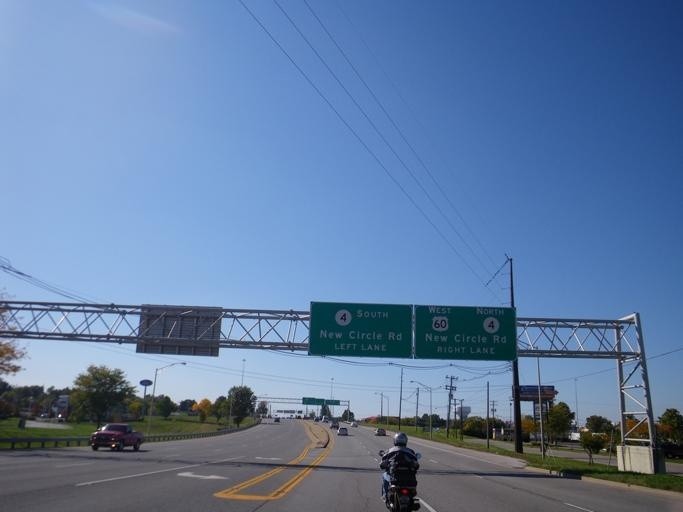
337;427;348;436
374;428;386;436
350;422;358;428
273;413;320;422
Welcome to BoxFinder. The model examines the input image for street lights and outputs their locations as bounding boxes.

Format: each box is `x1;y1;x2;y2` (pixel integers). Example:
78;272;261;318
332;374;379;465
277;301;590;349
146;362;187;436
374;392;390;428
26;396;34;418
330;376;334;400
240;358;246;388
410;380;433;440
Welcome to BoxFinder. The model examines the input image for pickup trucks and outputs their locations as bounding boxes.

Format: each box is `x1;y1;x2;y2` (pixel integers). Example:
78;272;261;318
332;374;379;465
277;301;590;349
88;424;144;452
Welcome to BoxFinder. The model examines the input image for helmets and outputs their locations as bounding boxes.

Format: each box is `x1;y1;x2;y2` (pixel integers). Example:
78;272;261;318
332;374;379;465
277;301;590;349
393;432;408;446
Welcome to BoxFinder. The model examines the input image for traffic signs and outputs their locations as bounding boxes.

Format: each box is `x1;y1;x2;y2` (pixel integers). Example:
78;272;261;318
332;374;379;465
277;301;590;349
511;384;559;401
308;301;414;358
413;305;517;363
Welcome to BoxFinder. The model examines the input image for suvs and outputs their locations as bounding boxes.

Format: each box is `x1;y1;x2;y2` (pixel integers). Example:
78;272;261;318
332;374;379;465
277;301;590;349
329;420;339;429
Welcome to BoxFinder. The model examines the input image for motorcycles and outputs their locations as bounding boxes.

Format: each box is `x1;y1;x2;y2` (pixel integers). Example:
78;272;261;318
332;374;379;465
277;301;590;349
379;449;421;512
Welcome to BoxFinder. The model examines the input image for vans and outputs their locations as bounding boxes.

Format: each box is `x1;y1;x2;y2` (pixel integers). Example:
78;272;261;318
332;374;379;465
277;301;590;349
321;415;328;423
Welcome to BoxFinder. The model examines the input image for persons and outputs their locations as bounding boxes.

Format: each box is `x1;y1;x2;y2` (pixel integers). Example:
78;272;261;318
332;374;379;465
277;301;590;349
378;432;417;502
